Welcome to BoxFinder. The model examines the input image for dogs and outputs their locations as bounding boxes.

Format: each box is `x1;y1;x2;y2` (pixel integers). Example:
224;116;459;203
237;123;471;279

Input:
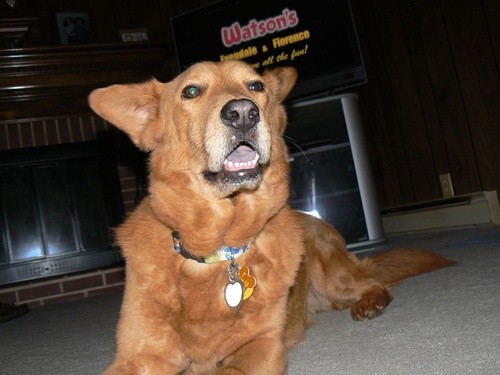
88;60;459;375
